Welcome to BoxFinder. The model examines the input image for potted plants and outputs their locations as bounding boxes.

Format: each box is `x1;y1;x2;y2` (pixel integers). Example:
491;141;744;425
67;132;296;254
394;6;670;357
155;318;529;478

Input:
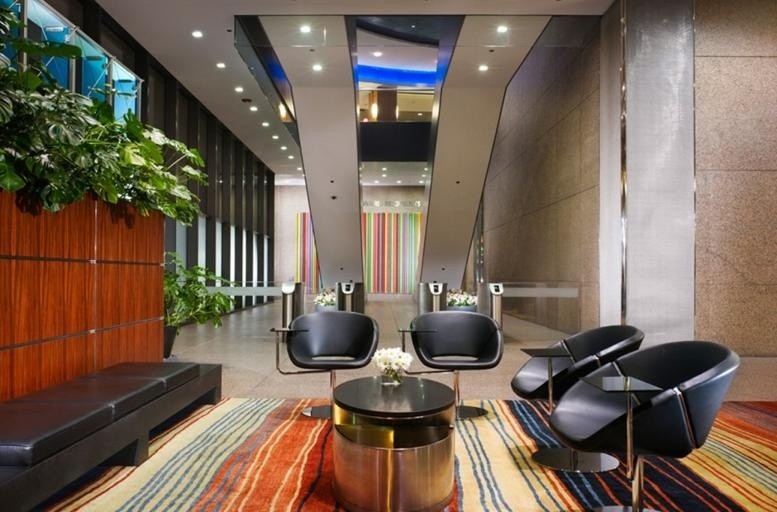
164;251;236;359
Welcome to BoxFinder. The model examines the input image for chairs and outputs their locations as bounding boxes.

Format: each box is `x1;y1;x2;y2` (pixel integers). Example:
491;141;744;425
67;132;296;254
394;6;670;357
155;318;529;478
400;311;503;417
549;341;741;512
267;310;379;418
510;325;644;473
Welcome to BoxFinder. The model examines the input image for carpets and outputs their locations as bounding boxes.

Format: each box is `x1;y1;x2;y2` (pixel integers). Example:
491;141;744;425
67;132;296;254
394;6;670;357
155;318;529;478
34;397;777;512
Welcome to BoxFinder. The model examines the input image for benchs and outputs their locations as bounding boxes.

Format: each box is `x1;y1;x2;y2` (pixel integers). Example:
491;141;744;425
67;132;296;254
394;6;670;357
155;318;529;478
0;361;222;512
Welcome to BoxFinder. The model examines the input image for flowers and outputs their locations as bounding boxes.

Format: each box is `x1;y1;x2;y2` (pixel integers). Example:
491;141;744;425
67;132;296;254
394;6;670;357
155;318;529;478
313;289;336;308
447;290;478;307
371;346;412;376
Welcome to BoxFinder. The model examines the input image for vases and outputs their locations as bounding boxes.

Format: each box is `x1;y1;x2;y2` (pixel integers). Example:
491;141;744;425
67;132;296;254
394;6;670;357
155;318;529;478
314;304;335;310
449;307;476;311
379;370;400;388
